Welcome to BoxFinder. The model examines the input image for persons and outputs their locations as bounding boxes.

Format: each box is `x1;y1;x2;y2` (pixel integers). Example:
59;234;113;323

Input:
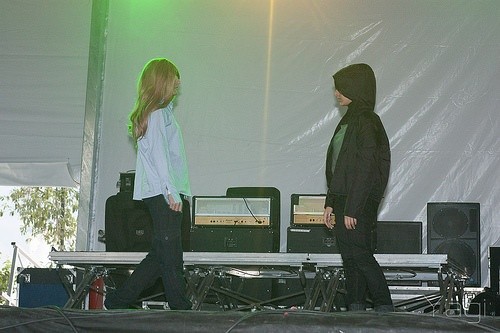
323;62;394;313
102;59;192;311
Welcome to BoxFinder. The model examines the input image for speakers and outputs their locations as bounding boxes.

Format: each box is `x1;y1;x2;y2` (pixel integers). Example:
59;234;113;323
287;226;374;254
375;222;423;255
426;202;481;286
106;194;189;254
189;227;279;252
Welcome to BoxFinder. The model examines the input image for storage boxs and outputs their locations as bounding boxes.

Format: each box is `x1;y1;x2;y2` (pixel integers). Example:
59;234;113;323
15;267;105;313
